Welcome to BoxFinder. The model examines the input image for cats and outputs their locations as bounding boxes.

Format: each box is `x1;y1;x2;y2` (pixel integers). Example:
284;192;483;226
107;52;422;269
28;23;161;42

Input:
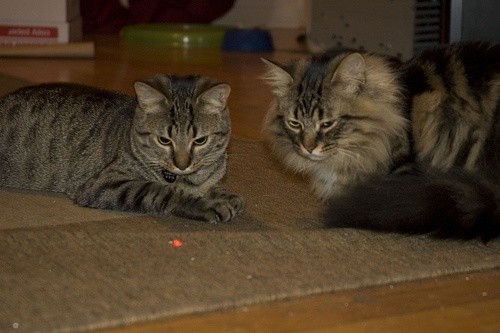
0;73;247;224
254;38;500;245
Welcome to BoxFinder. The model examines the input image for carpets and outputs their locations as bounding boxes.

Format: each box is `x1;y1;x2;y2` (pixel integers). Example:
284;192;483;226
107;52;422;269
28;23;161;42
0;140;500;333
